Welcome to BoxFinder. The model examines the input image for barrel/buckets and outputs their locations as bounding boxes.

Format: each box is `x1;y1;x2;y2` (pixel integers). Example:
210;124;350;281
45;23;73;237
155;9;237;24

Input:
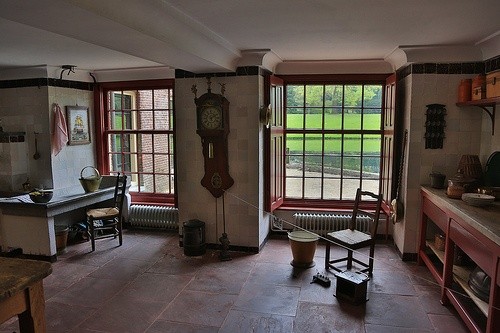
288;232;319;264
430;174;446;189
54;225;69;250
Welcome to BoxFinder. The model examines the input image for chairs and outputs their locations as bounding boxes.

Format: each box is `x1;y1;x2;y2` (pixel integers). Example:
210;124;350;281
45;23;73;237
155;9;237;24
325;188;383;277
86;172;127;252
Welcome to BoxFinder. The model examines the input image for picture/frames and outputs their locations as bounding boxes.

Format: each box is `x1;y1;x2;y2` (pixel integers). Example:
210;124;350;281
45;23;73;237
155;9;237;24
66;105;92;145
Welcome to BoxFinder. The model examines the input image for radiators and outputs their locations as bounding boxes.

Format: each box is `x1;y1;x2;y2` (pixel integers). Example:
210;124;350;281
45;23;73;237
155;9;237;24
294;215;373;237
129;205;179;232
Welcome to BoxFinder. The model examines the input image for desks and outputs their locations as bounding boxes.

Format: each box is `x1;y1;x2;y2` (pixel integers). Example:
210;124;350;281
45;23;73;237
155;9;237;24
0;176;132;260
0;257;53;333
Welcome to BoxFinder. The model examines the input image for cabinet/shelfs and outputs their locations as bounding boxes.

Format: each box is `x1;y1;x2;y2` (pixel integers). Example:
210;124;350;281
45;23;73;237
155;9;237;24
418;183;500;333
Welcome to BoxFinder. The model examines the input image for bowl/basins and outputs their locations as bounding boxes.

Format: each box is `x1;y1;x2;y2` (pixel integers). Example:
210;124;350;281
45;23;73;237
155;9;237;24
462;193;495;205
30;191;53;203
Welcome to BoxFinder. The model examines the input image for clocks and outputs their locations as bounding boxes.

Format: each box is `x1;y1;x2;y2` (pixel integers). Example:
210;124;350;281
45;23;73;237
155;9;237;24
202;107;221;130
